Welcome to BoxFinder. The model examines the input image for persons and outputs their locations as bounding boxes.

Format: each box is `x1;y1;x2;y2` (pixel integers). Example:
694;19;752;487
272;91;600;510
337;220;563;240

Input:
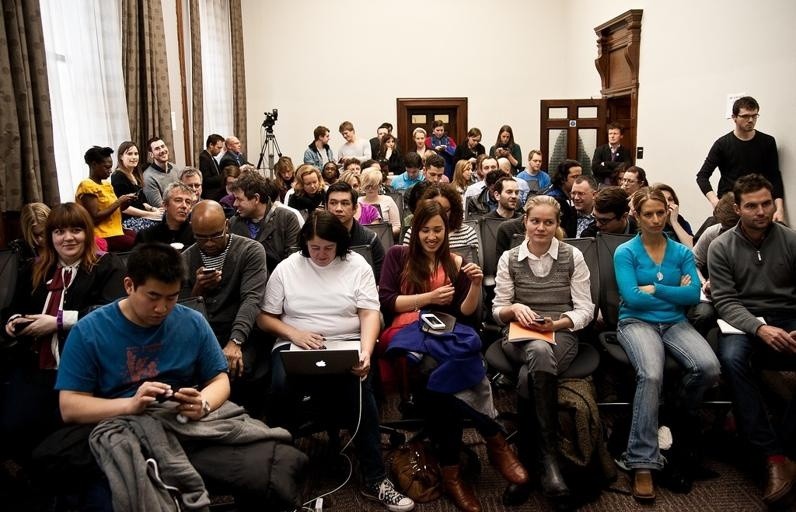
0;120;796;512
696;96;786;224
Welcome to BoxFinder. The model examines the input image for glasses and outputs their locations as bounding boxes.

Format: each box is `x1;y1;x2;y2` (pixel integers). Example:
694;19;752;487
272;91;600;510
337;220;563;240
736;113;759;120
192;228;227;244
365;185;379;190
590;212;624;226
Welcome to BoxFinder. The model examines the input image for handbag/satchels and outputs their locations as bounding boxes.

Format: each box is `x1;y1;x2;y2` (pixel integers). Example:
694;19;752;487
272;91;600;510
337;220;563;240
381;441;447;503
562;371;634;512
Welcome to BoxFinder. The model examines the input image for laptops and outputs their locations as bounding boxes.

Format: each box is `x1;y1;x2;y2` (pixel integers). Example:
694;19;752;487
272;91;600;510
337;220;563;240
279;340;362;375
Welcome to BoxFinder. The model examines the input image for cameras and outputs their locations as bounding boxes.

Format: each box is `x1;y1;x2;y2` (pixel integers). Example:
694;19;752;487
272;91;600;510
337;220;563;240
263;109;278;127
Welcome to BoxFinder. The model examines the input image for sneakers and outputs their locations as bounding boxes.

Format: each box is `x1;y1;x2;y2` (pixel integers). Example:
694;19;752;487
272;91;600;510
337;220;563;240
359;475;416;512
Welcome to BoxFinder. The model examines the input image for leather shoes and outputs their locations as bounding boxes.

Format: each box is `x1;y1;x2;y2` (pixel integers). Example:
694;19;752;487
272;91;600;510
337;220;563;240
761;456;796;504
631;469;657;502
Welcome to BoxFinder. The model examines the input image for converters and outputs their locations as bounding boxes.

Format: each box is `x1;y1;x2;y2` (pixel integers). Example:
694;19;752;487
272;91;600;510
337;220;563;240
314;498;323;512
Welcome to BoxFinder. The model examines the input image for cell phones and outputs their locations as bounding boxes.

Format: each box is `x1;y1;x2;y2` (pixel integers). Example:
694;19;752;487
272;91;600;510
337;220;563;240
156;393;186;403
534;319;545;324
13;321;34;335
203;268;216;275
421;314;445;330
606;334;617;344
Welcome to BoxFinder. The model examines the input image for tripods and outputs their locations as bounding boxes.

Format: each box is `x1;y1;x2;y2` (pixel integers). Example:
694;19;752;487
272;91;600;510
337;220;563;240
257;132;283;179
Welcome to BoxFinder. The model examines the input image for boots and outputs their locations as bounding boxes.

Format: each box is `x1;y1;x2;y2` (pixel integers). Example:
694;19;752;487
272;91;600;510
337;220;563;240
439;463;484;512
501;396;533;505
486;435;531;486
527;372;571;507
